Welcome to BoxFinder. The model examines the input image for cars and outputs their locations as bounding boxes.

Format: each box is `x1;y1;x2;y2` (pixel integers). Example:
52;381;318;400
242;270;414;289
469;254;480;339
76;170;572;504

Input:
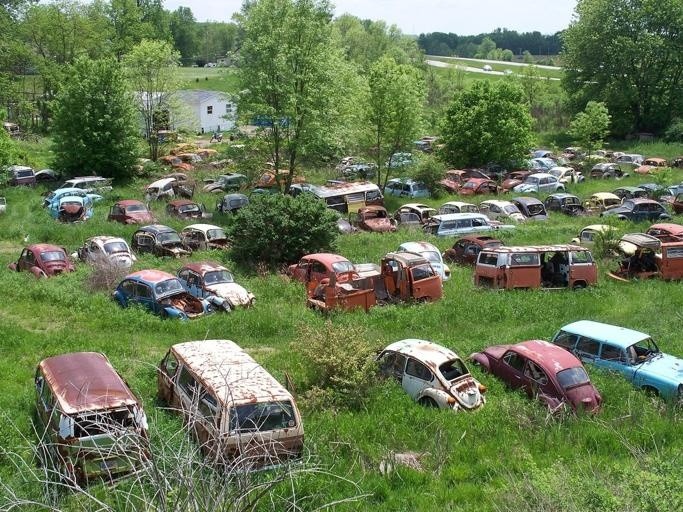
441;235;505;265
570;224;621;255
286;241;452;319
373;339;487;417
110;269;216;321
503;68;513;75
131;225;193;259
482;64;493;73
602;222;682;285
467;340;603;418
178;223;235;253
8;244;76;278
551;320;682;404
70;236;136;272
176;262;256;313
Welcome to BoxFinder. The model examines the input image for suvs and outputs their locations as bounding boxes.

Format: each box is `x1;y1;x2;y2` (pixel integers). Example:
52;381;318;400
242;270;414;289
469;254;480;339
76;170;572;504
1;122;682;238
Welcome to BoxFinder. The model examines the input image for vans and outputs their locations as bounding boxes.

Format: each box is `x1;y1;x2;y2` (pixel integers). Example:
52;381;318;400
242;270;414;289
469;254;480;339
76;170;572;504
156;340;304;478
33;350;151;490
474;245;599;292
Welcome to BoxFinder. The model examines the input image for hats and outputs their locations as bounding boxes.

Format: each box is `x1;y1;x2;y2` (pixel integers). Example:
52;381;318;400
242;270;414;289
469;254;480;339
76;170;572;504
482;64;493;73
503;68;513;75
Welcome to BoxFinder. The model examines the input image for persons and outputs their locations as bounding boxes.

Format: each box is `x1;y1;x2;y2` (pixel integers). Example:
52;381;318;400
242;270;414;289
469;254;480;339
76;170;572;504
549;251;565;273
643;250;657;273
